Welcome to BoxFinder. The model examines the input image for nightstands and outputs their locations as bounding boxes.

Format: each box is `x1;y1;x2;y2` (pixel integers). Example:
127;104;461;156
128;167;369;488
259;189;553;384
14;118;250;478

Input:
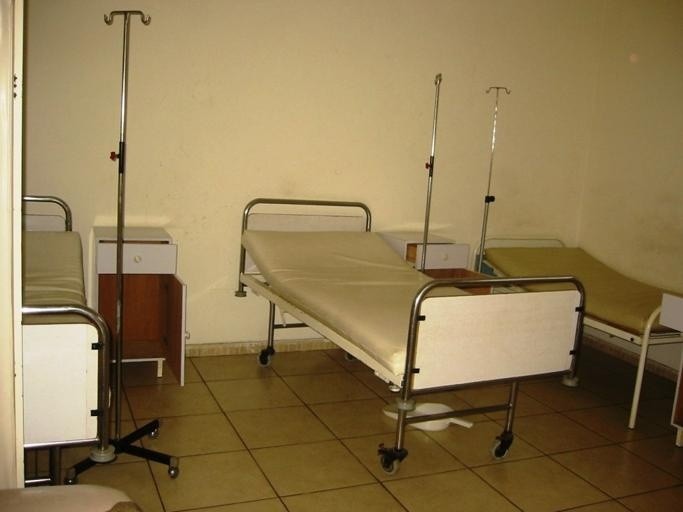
377;231;495;297
92;226;190;387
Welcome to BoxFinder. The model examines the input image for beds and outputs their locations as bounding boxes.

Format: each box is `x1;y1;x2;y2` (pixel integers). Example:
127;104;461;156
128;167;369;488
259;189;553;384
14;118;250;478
22;194;110;487
471;237;683;429
234;198;586;475
